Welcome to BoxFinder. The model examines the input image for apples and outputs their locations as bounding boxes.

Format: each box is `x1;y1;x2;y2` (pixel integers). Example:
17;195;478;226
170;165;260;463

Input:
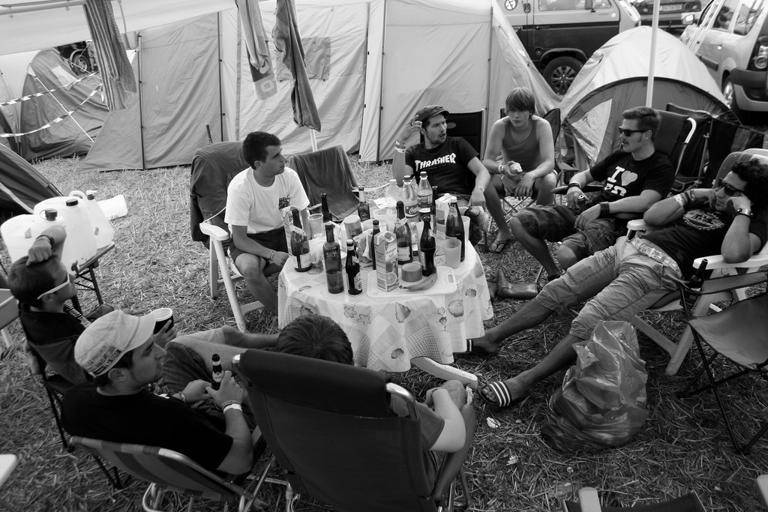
510;162;523;174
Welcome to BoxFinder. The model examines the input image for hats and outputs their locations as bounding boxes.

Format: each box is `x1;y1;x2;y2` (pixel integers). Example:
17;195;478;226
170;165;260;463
415;104;450;122
72;308;158;378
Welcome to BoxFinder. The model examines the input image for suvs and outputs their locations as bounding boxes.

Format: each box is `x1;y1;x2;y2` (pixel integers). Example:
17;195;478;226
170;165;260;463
636;0;701;39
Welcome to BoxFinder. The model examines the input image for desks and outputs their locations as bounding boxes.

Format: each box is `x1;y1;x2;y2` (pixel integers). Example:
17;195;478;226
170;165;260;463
70;241;116;315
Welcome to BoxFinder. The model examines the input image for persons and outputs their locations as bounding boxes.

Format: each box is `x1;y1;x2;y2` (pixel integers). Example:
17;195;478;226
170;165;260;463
479;86;557;256
8;224;178;399
272;312;469;497
222;130;312;318
55;307;279;480
469;157;767;412
509;104;676;279
392;105;492;244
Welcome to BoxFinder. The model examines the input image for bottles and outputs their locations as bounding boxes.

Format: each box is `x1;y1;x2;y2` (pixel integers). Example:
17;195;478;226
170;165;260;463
678;259;709;311
211;354;227;411
290;171;471;295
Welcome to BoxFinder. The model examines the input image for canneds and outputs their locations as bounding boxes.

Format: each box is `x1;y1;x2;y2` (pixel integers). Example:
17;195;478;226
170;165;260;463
574;195;586;215
343;215;363;239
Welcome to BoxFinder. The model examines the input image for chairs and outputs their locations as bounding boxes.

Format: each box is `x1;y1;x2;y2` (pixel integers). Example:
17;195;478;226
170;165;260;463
417;108;484;159
199;221;265;333
675;270;767;454
25;339;134;488
487;108;561;234
232;349;476;510
664;104;713;192
624;148;767;376
66;398;292;511
535;102;696;286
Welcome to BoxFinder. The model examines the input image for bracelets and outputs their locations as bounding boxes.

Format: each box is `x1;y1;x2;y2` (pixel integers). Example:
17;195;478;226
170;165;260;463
567;183;580;190
429;388;440;396
270;250;275;261
674;191;697;207
477;186;484;193
733;206;754;219
599;202;610;218
497;164;505;173
178;390;188;404
220;400;245;415
394;139;406;153
34;234;56;250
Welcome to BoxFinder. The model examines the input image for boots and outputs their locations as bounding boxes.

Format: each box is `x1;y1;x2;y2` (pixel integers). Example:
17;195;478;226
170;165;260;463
495;269;539;300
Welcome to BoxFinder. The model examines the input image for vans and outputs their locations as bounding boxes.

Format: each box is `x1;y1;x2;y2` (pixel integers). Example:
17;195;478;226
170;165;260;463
495;0;642;96
677;0;768;125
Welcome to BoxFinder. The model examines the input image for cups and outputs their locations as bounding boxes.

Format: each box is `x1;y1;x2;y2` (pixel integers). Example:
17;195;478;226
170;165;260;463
150;307;175;340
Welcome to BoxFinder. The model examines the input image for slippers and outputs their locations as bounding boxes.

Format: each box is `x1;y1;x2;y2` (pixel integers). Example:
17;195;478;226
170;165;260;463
476;379;532;406
452;338;498;358
489;228;514;254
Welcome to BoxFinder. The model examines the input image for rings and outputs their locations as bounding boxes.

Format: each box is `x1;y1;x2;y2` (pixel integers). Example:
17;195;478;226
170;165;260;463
410;122;414;127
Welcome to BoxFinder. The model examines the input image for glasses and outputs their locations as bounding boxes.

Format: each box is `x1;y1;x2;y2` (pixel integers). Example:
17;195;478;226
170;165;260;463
617;124;646;137
33;269;71;303
714;177;746;197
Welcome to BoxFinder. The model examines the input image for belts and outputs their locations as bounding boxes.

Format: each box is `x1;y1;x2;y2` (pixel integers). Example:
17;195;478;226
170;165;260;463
626;226;683;279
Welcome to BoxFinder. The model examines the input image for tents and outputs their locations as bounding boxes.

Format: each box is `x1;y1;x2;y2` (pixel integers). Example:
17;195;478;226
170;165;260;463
0;143;66;292
558;24;745;183
0;48;112;167
80;2;561;179
